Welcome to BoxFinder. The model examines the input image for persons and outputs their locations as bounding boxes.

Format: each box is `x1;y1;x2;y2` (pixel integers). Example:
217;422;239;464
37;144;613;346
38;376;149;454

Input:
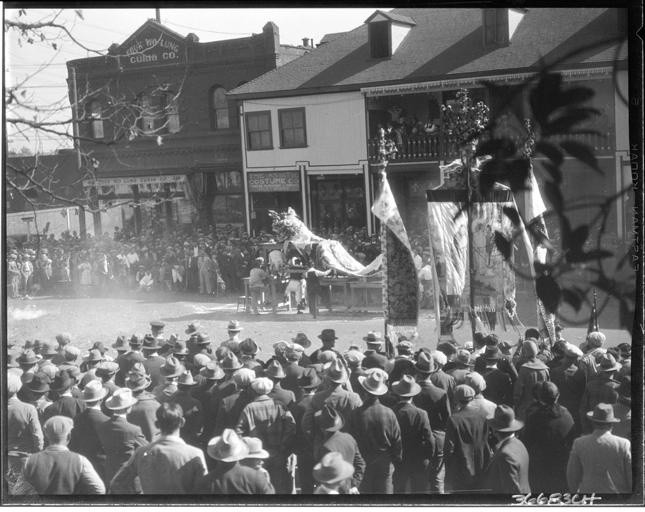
373;114;438;139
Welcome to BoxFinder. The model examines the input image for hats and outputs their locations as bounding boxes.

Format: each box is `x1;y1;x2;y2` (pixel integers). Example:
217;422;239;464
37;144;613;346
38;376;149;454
7;321;631;434
241;436;271;461
205;428;251;463
310;452;357;486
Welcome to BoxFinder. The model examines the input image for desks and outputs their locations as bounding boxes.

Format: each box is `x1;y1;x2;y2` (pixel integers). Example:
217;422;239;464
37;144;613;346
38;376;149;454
242;275;382;318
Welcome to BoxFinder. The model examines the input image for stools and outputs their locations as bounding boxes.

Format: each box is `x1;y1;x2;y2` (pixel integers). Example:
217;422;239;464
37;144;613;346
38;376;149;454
236;295;251;314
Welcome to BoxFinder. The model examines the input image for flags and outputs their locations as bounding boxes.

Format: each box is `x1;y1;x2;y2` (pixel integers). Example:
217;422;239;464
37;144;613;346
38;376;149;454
523;161;557;346
370;174;421;328
429;186;520;332
